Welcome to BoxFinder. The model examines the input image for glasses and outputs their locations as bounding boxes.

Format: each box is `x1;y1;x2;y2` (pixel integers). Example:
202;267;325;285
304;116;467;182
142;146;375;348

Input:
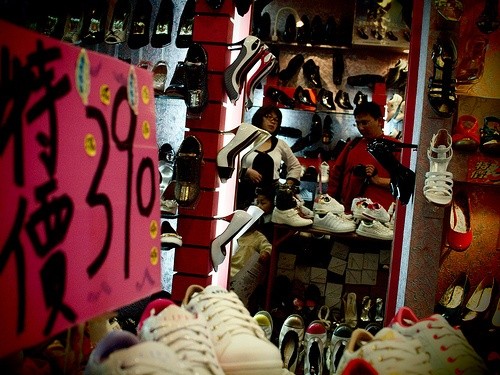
356;120;371;125
264;115;279;125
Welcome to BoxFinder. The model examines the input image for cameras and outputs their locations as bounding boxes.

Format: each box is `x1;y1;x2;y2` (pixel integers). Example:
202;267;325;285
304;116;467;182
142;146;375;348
352;165;367;176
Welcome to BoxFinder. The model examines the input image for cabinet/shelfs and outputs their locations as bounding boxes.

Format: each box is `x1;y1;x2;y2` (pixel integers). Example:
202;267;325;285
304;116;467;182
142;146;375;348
256;41;402;116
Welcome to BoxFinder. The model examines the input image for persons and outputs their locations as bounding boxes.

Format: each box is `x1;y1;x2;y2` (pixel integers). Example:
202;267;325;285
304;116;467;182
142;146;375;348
326;102;402;213
255;187;276;238
235;106;302;212
228;219;273;284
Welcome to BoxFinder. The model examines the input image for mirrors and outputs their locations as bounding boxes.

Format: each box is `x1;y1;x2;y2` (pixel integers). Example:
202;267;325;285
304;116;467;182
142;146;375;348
226;0;423;375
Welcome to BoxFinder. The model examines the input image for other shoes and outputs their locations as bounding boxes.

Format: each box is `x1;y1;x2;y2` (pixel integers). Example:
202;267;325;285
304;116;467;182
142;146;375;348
2;3;497;373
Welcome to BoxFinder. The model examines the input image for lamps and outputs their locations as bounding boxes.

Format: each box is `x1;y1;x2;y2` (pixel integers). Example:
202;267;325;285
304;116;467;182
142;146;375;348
273;6;303;41
